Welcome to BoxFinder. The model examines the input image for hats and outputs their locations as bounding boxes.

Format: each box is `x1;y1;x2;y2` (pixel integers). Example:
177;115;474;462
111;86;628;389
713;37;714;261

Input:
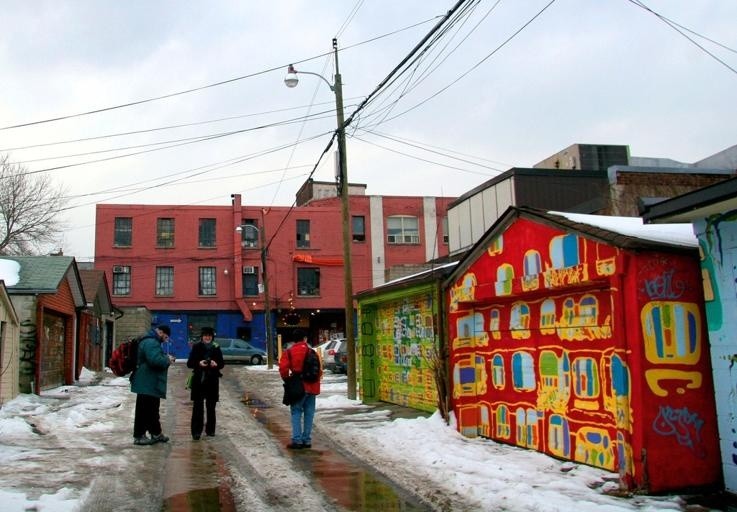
201;327;214;334
158;325;170;336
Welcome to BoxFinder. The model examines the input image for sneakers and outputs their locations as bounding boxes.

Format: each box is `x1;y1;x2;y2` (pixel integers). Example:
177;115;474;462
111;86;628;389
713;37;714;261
133;436;154;444
208;433;215;436
286;443;301;449
192;433;200;440
151;434;169;442
302;444;311;448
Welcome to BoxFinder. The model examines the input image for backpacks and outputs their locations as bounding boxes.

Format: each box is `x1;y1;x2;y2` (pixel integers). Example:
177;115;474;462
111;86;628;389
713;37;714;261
108;335;157;377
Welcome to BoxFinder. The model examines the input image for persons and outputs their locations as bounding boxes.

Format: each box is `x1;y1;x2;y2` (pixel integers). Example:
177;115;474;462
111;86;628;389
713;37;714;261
278;327;321;450
187;326;224;441
130;324;176;446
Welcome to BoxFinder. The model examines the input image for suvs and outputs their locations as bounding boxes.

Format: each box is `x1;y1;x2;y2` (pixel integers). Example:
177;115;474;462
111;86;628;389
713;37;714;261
310;337;359;376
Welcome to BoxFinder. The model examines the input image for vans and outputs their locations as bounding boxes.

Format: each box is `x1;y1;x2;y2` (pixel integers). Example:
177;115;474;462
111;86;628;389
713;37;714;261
213;337;268;365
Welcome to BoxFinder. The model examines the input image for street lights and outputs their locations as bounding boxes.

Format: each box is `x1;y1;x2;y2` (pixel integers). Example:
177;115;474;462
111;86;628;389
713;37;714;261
235;223;273;370
283;62;358;402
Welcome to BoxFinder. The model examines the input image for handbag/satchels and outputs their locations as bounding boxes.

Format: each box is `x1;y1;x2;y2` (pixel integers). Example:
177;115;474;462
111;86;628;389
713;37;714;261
303;348;320;383
283;372;305;406
184;374;192;390
